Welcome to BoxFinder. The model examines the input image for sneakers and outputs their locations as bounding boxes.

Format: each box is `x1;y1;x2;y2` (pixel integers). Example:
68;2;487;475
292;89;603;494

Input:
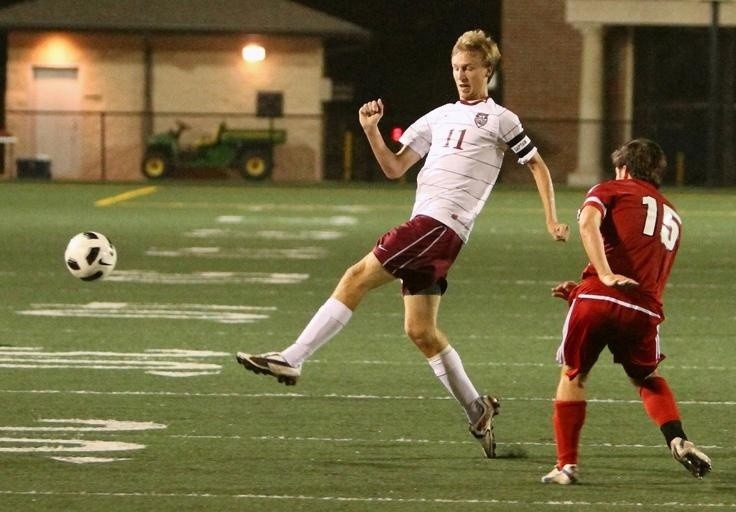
468;395;500;458
541;463;577;485
236;352;301;385
670;437;712;480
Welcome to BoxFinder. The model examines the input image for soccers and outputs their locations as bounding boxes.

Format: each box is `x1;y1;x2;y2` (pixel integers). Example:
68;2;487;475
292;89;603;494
64;232;117;282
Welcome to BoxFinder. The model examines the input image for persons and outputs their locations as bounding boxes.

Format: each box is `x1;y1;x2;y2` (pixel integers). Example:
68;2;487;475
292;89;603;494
235;29;570;460
539;138;713;486
